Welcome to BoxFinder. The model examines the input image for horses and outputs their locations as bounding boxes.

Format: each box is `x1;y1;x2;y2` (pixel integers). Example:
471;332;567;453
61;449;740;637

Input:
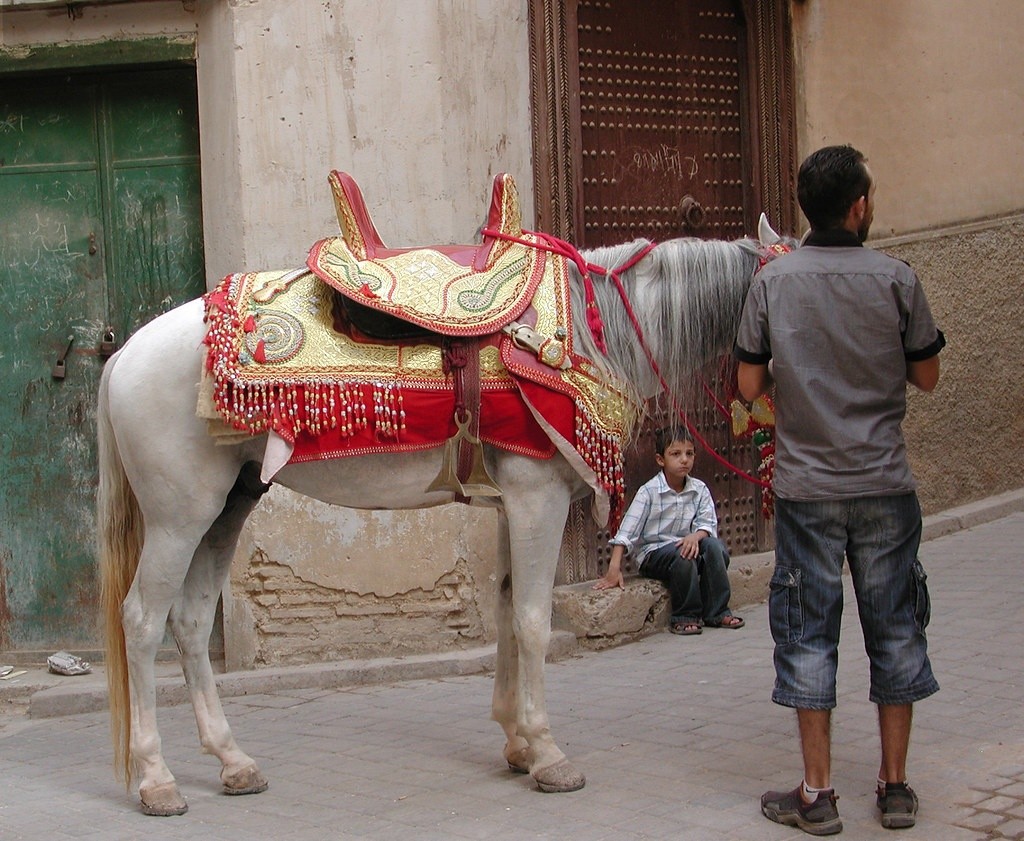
93;207;814;818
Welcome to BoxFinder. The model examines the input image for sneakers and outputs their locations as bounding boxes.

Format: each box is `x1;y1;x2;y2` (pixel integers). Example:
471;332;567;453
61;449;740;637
875;781;919;827
761;779;843;835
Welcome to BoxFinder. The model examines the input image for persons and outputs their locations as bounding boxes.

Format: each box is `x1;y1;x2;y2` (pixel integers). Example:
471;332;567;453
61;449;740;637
733;145;947;835
592;426;745;634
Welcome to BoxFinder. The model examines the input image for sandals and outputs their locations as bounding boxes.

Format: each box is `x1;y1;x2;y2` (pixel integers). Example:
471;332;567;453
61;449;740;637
669;620;703;634
702;615;745;628
